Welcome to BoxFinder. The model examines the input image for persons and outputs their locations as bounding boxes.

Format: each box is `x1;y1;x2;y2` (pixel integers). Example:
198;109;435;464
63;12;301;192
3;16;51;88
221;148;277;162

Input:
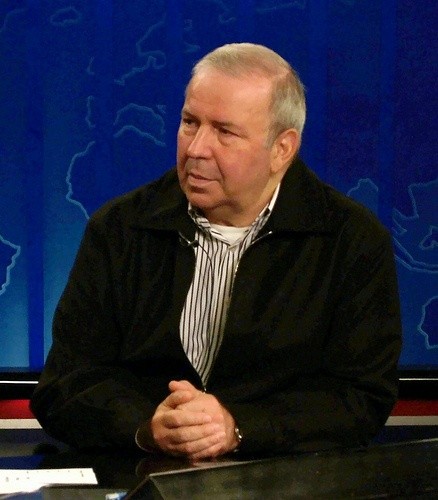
29;43;405;462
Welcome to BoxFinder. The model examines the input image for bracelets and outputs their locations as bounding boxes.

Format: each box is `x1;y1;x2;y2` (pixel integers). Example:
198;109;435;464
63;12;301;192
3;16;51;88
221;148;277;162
232;422;243;456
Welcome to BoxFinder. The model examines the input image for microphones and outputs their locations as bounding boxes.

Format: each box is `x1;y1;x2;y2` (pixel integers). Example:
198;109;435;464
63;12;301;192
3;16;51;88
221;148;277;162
179;231;199;248
